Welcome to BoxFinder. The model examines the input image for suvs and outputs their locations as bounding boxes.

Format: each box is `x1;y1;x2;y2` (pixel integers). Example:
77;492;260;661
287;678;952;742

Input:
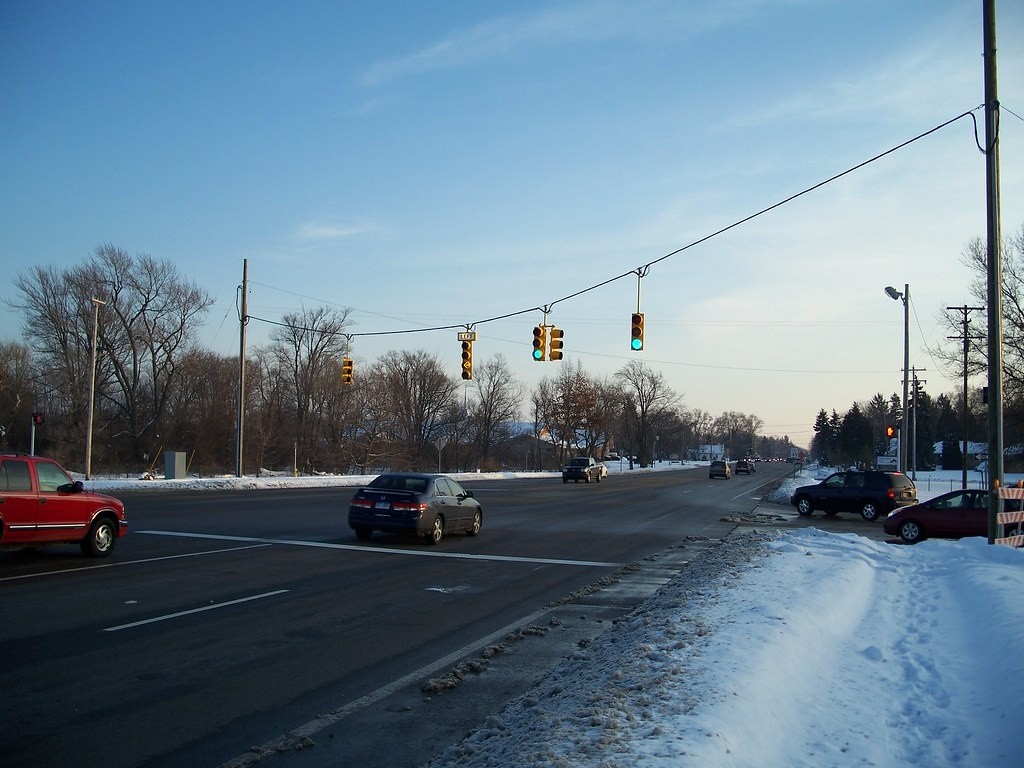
791;468;920;522
0;451;129;558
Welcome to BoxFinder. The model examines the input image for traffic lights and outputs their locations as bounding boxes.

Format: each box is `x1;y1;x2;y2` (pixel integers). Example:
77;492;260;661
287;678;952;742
461;341;473;380
341;357;354;385
532;326;547;361
631;312;645;350
886;426;895;437
34;411;43;425
549;327;564;361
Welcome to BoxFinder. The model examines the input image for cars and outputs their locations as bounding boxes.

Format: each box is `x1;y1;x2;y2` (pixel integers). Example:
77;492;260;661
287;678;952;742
348;471;483;545
709;460;731;479
562;457;608;483
735;460;757;475
884;489;1024;545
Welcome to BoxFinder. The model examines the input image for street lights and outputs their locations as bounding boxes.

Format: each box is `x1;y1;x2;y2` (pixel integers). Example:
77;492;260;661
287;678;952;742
87;297;106;481
884;283;910;474
